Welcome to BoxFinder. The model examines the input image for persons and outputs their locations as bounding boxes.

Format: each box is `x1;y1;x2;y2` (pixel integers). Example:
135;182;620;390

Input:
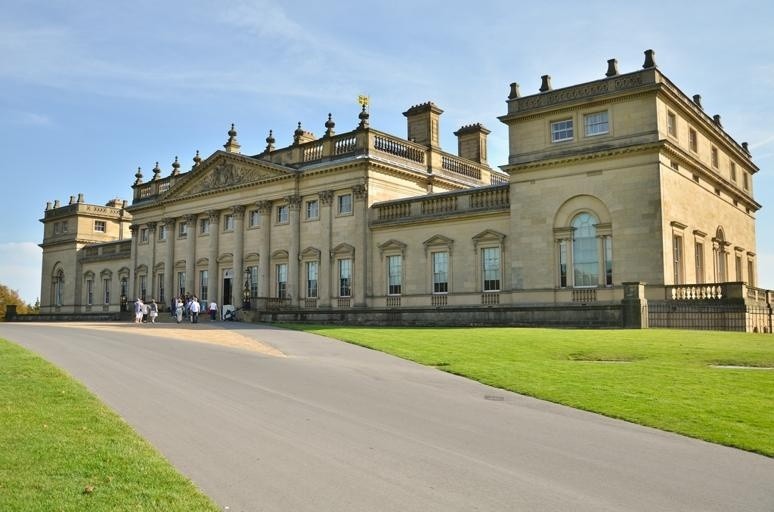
170;291;201;324
140;302;151;323
208;298;219;322
148;299;159;323
134;299;143;324
